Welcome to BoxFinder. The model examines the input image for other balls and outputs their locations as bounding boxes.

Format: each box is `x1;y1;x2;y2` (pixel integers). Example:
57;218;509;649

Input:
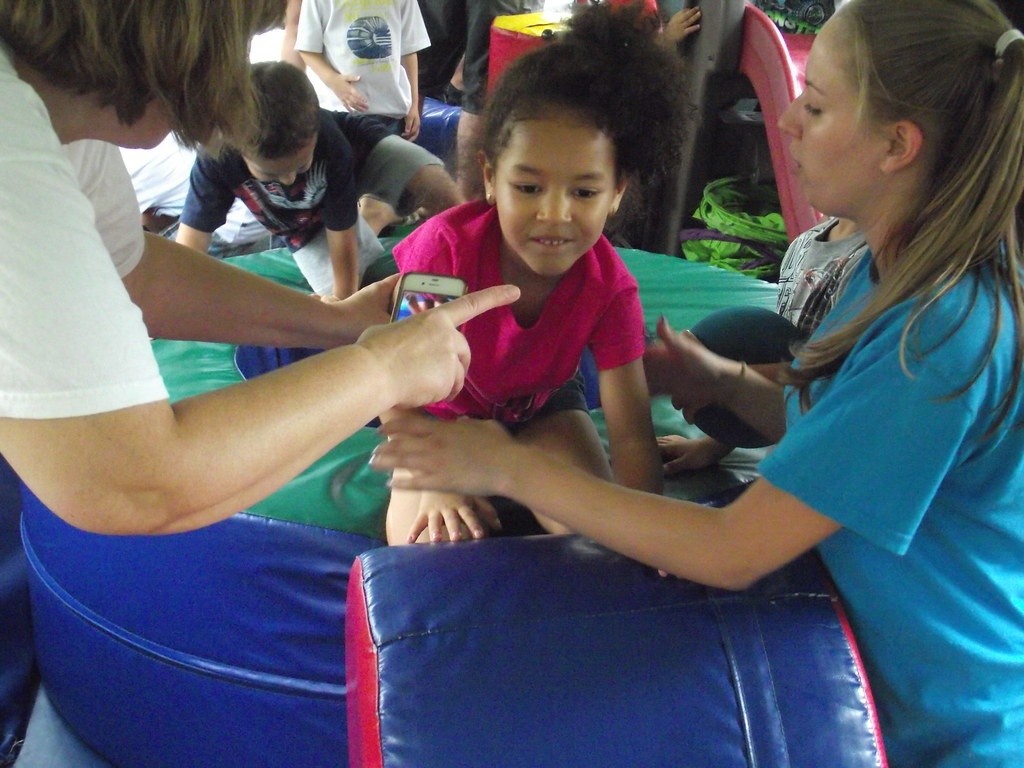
690;305;803;449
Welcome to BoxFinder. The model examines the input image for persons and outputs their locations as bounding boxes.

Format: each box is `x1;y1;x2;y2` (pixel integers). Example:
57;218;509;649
386;0;673;588
0;0;522;539
572;0;702;56
279;0;546;144
375;0;1021;767
176;59;471;306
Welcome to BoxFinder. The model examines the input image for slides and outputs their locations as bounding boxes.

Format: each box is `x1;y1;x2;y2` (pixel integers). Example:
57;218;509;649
738;2;824;244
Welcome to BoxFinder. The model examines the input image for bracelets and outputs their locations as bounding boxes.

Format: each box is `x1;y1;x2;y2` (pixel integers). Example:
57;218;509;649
729;359;745;412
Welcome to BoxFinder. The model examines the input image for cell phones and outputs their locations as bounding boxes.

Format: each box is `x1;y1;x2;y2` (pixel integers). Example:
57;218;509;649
388;270;469;331
